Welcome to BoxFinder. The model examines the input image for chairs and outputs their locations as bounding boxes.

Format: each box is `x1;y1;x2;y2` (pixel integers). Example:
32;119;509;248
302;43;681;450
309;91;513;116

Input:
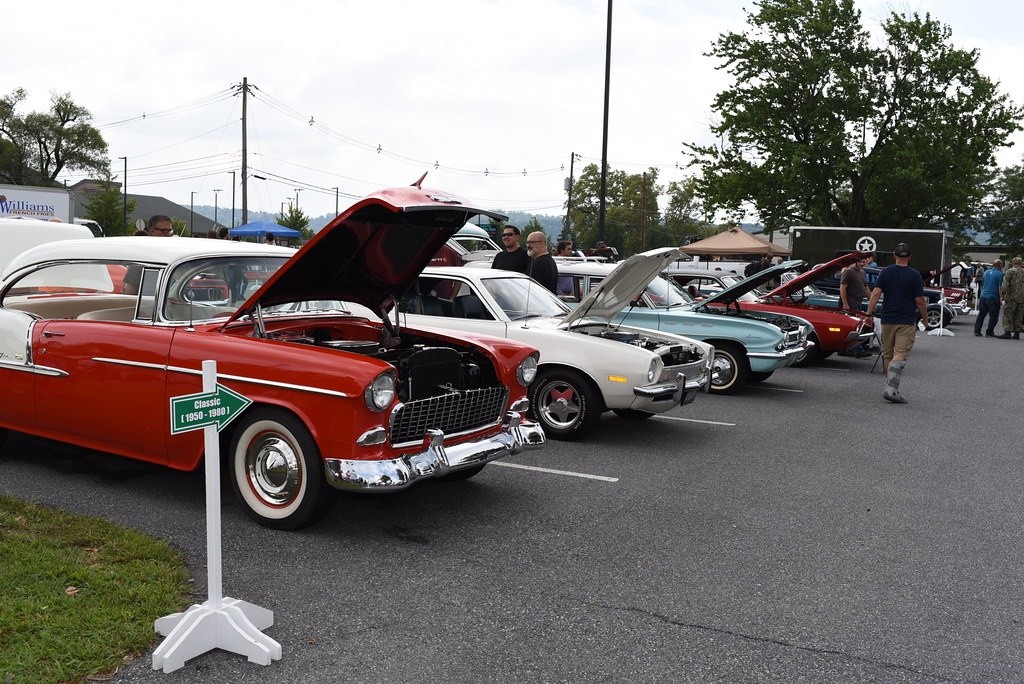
688;286;701;297
408;295;487;319
869;317;885;373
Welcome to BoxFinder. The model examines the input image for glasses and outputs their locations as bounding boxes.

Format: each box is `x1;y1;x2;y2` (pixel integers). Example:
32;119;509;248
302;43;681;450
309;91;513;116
503;233;517;237
601;247;605;248
153;227;174;234
526;241;541;245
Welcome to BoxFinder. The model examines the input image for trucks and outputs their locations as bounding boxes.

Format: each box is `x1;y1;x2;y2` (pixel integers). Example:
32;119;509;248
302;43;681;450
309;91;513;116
0;183;108;238
951;262;1004;289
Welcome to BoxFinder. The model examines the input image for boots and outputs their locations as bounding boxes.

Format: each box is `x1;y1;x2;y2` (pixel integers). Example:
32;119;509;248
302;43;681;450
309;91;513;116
883;358;908;403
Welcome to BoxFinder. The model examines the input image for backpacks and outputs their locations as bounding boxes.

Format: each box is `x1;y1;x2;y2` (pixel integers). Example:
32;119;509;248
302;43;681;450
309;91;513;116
744;262;757;278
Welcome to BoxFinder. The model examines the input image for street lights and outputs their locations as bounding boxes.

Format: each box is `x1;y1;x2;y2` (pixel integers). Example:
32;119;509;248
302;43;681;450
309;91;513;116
280;201;287;222
331;187;338;218
213;189;223;224
293;188;305;213
228;171;235;229
190;191;197;237
118;156;128;235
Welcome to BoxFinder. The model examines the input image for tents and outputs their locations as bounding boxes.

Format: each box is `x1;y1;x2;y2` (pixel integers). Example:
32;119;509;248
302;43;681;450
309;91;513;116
677;226;792;285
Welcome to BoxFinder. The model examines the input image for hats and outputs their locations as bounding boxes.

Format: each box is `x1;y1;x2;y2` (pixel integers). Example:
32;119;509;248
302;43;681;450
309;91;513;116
895;243;912;257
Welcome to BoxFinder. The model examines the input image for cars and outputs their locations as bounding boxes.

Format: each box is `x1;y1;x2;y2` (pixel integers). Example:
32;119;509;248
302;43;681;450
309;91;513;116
278;245;715;442
636;249;875;367
682;271;883;321
0;186;549;529
545;259;817;396
34;221;506;307
811;265;958;331
564;246;754;282
922;261;971;316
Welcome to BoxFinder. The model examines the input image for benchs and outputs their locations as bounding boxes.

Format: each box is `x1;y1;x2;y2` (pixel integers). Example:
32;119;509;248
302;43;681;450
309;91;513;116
5;296;238;322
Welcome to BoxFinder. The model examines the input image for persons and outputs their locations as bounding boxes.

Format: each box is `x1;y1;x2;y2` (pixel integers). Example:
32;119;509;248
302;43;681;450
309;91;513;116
839;255;877;358
960;257;1024;340
688;286;709;300
551;239;615;296
865;242;928;403
122;216;175;295
208;228;276;306
524;231;558;296
491;225;532;276
744;253;784;293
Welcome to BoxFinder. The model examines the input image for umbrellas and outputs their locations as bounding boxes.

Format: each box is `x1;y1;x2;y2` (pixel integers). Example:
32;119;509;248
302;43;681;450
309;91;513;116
228;220;301;285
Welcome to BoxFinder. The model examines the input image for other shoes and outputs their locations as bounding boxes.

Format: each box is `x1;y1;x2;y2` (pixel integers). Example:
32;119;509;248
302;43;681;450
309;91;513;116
1013;334;1019;339
238;295;246;301
837;350;872;358
986;332;999;338
998;334;1011;339
974;332;983;336
